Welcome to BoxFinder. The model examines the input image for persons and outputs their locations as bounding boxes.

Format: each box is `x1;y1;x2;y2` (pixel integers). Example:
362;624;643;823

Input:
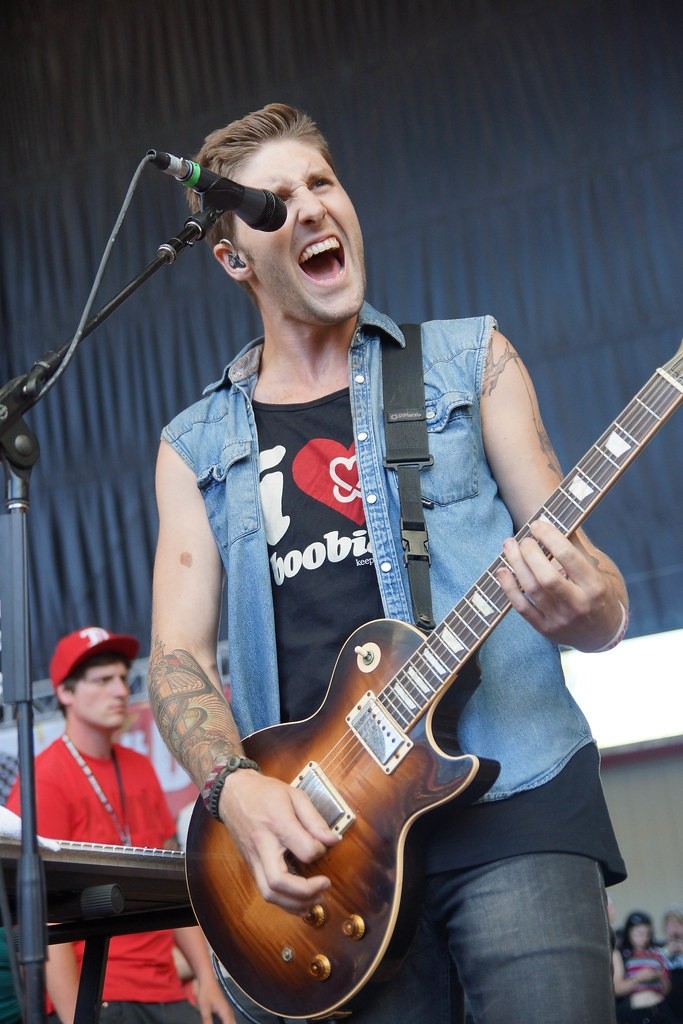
607;894;683;1024
149;103;628;1024
0;626;237;1024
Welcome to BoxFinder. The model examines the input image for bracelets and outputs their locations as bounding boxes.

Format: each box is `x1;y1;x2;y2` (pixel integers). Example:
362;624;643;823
209;757;261;824
594;600;629;653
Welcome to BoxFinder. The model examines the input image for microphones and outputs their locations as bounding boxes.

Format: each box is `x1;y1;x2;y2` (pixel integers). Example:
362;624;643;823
147;149;287;232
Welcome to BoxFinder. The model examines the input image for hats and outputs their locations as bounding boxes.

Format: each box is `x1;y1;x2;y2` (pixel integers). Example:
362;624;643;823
51;627;140;686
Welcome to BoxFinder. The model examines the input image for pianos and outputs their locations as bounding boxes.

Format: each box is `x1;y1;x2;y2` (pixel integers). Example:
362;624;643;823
0;835;196;1024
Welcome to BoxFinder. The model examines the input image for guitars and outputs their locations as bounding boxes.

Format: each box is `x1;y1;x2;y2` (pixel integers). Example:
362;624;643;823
184;345;683;1019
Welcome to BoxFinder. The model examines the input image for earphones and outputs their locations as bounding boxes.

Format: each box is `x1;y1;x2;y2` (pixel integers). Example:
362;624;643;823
227;252;246;269
